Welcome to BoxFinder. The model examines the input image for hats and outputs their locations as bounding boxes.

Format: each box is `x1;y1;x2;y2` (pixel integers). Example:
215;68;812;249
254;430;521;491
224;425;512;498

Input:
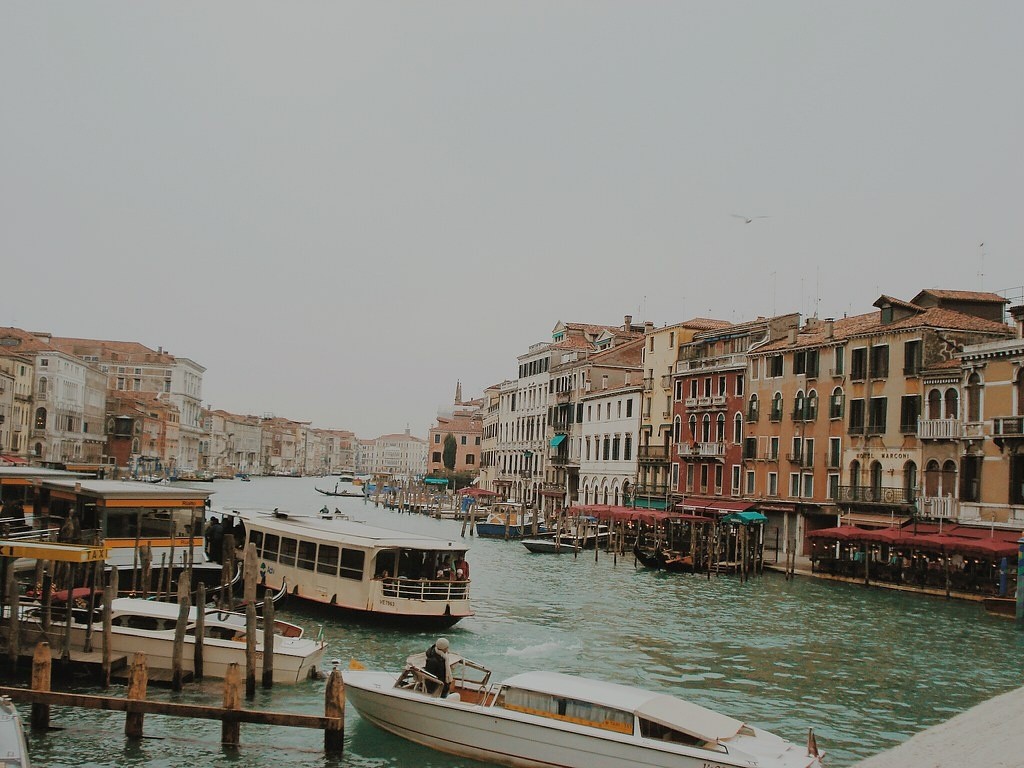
456;569;463;574
435;637;450;649
437;570;443;577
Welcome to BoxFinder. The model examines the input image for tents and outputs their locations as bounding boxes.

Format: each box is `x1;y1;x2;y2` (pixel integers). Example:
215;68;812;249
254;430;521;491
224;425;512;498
806;522;1024;597
455;489;501;515
554;504;767;587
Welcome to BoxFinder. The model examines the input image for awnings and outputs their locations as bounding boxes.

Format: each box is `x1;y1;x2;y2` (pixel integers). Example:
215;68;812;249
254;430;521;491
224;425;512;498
1;455;32;465
705;501;756;515
840;513;912;529
549;435;566;447
676;499;717;513
625;499;671;510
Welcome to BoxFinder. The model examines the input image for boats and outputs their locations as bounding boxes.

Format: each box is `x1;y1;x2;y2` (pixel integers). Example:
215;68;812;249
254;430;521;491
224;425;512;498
338;468;355;482
521;539;582;555
314;486;366;497
203;506;476;629
0;694;31;768
632;539;708;574
360;470;492;519
325;648;830;768
4;587;329;686
553;515;617;546
0;450;290;617
474;501;546;538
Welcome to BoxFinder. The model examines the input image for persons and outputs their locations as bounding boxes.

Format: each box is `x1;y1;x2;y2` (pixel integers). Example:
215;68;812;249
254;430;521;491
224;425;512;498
426;638;453;698
320;505;329;519
205;516;469;599
335;508;341;513
334;482;339;493
